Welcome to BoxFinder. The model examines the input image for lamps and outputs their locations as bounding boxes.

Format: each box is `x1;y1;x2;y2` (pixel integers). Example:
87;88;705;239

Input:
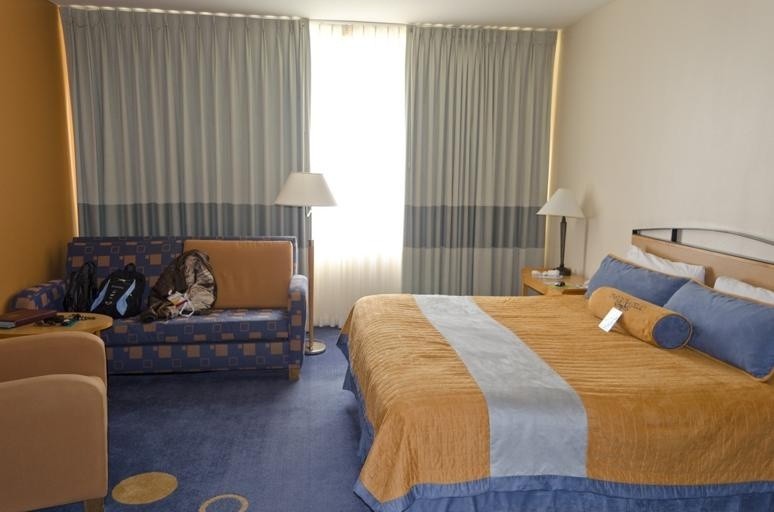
275;170;337;355
535;187;584;277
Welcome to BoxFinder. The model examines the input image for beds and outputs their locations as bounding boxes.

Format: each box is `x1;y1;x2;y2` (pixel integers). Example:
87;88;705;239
328;226;773;511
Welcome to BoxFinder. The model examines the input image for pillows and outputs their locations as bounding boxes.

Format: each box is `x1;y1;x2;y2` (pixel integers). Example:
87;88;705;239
581;254;691;308
713;274;773;308
582;284;695;350
622;242;705;286
659;277;774;383
178;239;294;313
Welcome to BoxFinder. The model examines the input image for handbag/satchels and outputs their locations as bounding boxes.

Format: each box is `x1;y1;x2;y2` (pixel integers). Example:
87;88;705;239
88;270;145;319
63;272;96;312
148;249;217;316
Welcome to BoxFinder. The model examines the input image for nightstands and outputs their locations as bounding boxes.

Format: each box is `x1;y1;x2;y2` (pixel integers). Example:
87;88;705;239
517;262;590;300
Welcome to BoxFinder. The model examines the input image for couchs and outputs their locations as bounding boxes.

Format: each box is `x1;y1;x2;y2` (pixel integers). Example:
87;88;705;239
7;232;308;382
0;328;109;512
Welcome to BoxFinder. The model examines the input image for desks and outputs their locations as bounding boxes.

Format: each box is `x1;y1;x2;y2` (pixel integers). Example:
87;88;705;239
0;310;113;343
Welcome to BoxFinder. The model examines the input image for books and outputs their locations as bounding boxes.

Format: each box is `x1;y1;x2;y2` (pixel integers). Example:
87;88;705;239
0;309;57;328
